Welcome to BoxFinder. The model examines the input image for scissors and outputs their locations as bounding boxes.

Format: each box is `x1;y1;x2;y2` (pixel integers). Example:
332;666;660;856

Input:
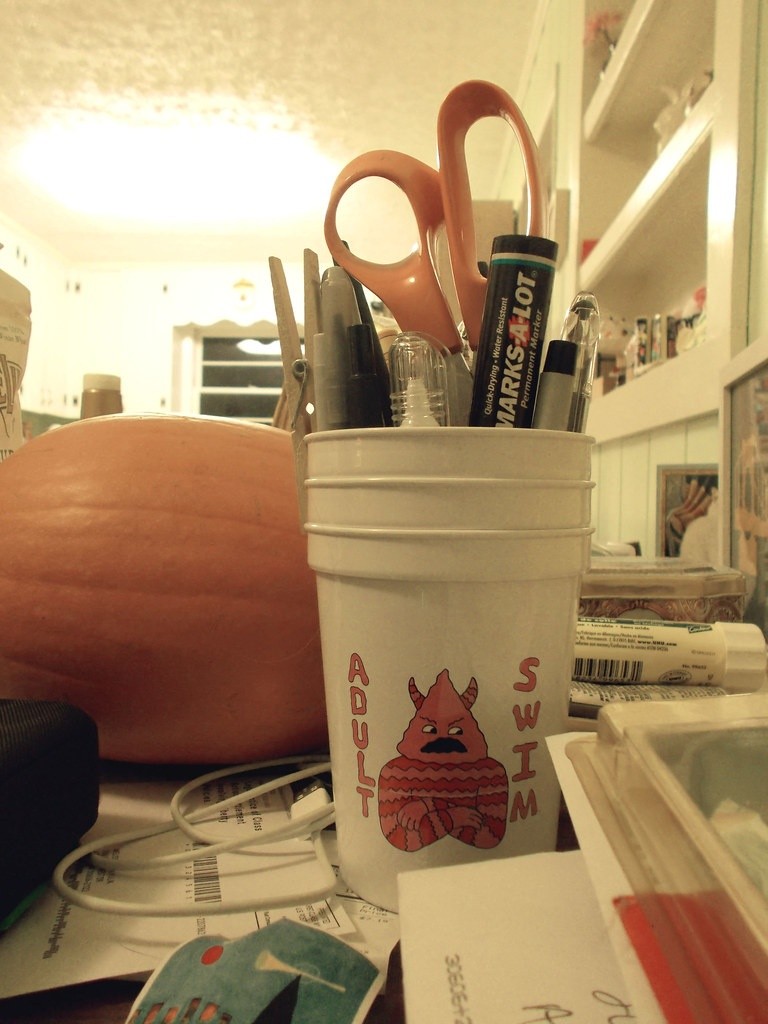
323;78;544;429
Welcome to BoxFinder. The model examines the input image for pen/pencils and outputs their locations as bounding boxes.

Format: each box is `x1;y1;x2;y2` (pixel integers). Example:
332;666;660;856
310;239;601;434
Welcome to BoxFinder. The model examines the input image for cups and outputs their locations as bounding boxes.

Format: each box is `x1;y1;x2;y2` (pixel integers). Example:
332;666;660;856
81;372;122;419
304;425;593;913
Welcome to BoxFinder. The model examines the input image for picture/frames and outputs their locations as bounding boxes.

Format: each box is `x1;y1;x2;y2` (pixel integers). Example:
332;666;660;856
716;331;768;646
656;462;722;569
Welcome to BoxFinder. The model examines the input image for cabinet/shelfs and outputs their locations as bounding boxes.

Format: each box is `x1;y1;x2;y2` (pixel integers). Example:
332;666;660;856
562;0;760;568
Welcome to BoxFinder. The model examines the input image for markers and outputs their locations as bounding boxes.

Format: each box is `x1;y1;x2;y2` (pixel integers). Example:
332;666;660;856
466;234;579;432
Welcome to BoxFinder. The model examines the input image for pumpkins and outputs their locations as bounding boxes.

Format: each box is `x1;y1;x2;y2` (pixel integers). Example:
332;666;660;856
0;415;328;766
